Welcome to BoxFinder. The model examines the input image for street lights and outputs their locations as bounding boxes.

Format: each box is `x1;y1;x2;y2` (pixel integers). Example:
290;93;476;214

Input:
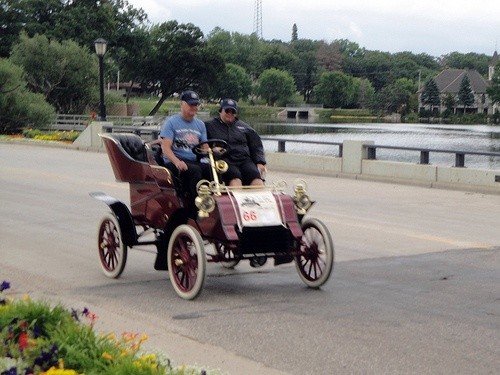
94;37;108;122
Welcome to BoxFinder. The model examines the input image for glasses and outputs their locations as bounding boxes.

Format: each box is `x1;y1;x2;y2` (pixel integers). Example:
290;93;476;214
225;108;235;114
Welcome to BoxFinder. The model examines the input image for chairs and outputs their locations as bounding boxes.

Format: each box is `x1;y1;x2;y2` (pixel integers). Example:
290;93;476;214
98;132;172;184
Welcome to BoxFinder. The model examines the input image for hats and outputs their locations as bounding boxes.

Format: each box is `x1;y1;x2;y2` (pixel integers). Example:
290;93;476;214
220;98;237;114
180;91;201;105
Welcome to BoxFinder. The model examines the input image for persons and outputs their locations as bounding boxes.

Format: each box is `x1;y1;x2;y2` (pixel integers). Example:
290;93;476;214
203;98;267;190
160;91;222;221
